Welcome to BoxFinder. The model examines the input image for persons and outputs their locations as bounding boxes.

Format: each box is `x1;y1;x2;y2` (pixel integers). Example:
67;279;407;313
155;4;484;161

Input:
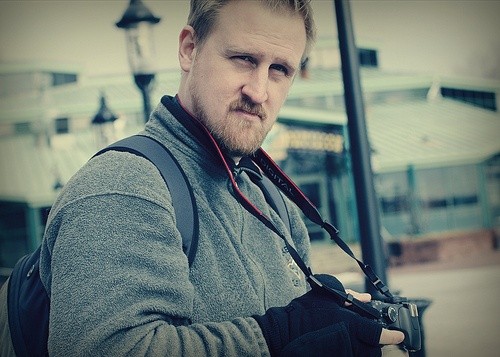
41;0;404;357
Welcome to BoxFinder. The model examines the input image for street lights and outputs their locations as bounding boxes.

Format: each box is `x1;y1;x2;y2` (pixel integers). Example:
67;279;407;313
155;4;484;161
89;96;121;148
113;0;163;128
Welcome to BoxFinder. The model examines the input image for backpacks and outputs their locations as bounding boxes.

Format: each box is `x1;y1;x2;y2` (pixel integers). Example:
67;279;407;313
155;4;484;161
0;134;199;357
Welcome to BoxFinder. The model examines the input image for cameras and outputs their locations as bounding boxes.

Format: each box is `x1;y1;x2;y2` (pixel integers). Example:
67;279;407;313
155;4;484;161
344;300;422;353
